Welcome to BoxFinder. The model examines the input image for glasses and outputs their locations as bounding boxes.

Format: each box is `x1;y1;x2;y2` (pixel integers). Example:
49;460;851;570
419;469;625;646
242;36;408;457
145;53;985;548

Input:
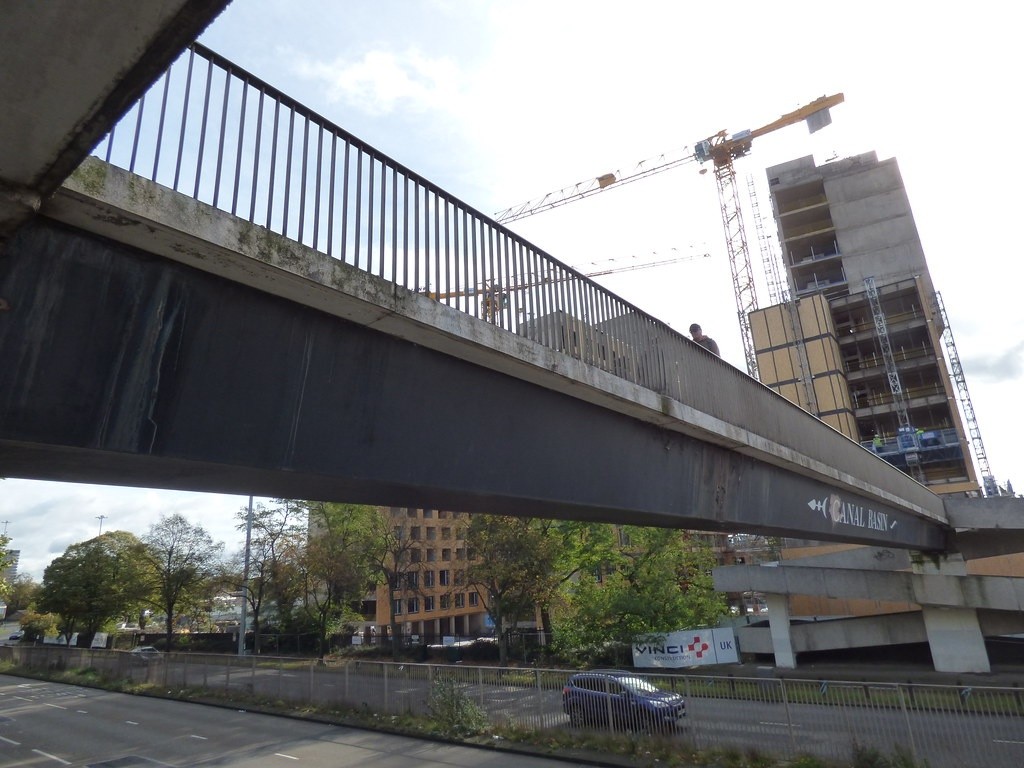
690;327;699;334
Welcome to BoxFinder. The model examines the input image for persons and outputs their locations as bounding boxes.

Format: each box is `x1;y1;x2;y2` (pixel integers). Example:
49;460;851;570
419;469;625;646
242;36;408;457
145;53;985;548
915;428;924;434
872;434;884;447
689;324;720;359
635;336;671;397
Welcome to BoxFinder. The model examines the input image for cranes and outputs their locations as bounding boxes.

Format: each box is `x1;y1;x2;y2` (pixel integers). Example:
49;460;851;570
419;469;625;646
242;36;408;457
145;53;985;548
417;239;715;327
493;89;846;383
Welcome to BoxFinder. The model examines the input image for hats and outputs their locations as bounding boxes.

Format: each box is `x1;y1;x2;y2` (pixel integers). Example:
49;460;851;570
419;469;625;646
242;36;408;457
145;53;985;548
690;324;700;331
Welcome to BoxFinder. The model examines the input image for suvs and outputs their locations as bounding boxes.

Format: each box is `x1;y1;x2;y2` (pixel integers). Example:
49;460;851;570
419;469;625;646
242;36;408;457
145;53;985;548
563;669;687;731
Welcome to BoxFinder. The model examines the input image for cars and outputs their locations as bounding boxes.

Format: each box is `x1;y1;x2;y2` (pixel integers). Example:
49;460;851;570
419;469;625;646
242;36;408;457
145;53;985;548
114;617;240;635
9;632;23;641
130;646;167;666
